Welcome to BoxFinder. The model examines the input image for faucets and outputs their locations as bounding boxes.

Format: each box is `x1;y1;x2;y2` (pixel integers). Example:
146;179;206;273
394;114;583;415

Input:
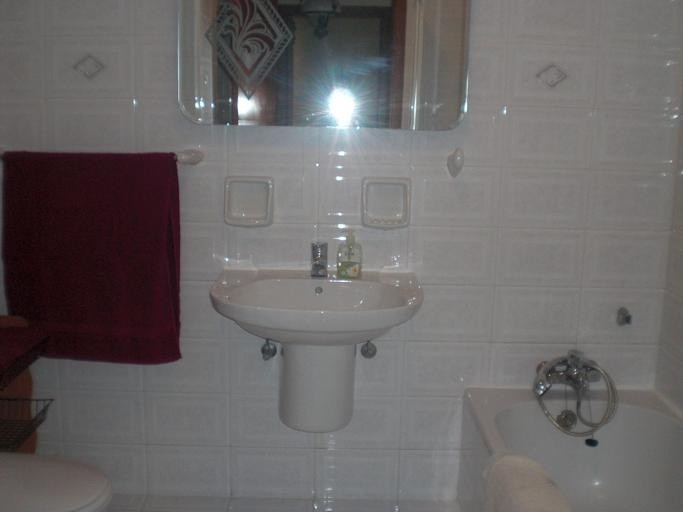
308;237;329;278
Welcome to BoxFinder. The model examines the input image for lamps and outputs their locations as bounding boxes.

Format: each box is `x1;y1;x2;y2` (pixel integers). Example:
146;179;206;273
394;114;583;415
295;1;344;39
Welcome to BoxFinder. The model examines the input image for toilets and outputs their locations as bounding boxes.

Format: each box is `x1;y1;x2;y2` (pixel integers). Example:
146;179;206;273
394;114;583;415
1;442;116;511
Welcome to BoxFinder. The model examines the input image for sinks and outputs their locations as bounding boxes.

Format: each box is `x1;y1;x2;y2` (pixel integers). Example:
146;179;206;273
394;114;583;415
210;269;423;345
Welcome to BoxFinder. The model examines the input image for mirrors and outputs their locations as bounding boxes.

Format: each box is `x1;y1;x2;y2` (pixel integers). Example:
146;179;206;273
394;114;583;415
168;1;476;133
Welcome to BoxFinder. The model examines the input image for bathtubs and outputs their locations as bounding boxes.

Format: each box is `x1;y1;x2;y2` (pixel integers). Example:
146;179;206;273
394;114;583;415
457;384;683;511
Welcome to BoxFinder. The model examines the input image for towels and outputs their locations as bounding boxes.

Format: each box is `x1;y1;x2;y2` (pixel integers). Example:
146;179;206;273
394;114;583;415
0;146;185;364
